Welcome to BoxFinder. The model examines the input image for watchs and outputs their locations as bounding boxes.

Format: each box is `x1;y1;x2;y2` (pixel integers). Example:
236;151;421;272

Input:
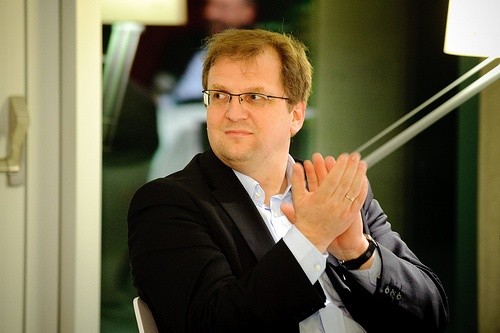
338;233;378;271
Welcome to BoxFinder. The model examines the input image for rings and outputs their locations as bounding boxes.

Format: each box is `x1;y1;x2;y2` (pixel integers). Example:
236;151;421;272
344;196;354;202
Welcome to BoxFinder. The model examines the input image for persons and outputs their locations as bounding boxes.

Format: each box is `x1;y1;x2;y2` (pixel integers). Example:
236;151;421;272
143;1;257;184
124;29;455;333
